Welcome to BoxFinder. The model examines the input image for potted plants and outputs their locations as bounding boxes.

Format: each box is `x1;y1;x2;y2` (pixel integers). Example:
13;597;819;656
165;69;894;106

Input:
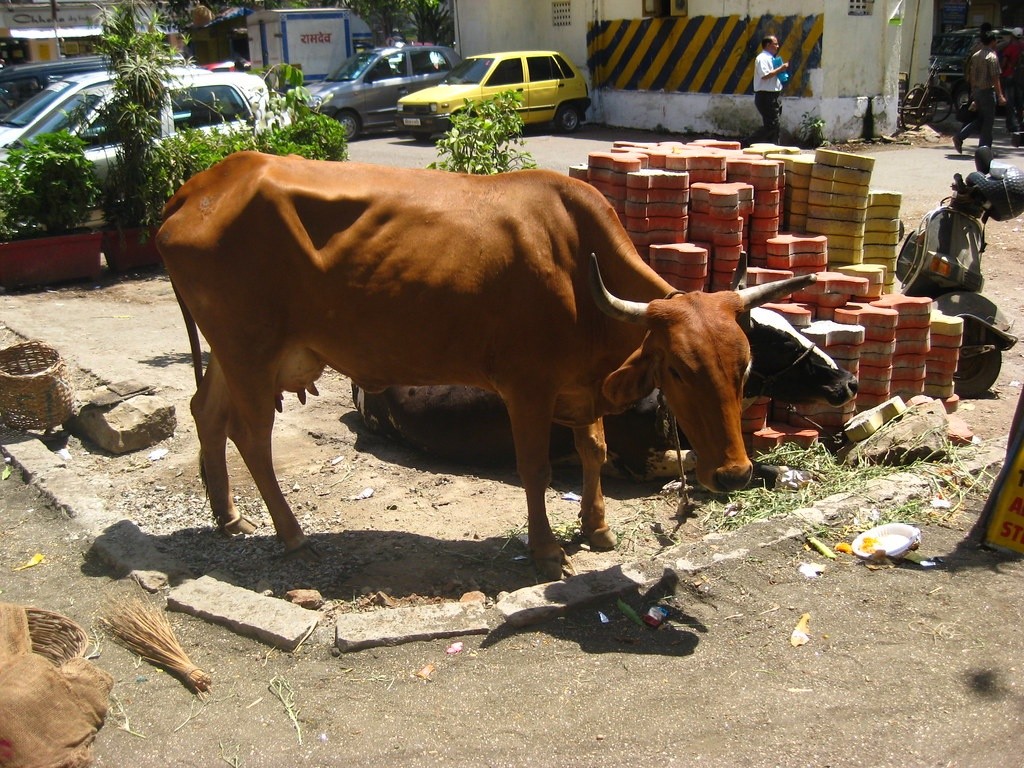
103;142;159;274
0;128;103;291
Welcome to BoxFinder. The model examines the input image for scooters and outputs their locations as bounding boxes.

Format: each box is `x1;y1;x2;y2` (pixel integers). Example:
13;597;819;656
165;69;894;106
895;145;1019;398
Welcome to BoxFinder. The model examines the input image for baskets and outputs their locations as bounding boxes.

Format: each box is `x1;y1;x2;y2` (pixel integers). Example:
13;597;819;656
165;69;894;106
0;339;76;430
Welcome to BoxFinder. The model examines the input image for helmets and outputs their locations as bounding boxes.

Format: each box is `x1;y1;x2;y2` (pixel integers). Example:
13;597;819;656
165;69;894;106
966;159;1024;221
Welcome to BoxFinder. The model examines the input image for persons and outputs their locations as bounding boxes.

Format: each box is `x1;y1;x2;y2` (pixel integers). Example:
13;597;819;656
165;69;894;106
952;22;1024;154
753;32;791;141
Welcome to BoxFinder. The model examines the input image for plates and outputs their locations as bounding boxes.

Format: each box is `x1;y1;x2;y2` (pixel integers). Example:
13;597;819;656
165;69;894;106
852;523;918;558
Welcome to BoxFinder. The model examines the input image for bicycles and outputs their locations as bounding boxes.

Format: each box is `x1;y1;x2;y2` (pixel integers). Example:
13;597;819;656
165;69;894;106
901;56;953;132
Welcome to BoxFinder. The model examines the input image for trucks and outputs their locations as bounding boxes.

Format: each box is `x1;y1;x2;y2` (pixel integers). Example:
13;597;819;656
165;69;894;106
247;7;375;98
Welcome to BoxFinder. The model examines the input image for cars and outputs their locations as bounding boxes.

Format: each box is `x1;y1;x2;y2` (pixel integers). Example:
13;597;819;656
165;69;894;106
299;46;464;141
394;50;591;143
932;28;1011;118
0;55;290;205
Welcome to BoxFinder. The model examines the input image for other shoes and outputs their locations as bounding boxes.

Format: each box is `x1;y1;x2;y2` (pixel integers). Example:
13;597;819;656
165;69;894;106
952;135;963;154
739;139;750;149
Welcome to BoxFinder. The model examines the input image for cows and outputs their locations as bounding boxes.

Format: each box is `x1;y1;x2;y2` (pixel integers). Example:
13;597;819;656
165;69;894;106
350;250;860;491
154;148;819;584
